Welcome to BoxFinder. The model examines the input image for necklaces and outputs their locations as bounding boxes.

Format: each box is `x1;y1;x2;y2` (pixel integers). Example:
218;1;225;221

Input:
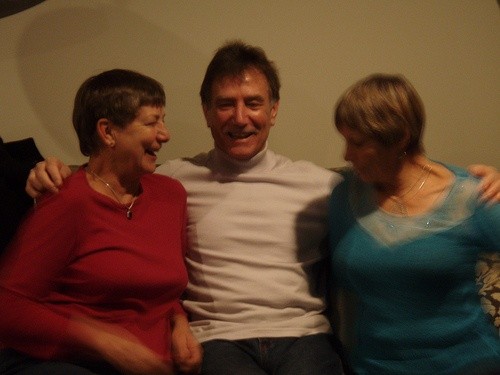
385;165;431;214
86;165;139;220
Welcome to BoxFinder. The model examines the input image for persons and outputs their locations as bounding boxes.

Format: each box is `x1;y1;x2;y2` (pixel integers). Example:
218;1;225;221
326;74;500;375
23;40;500;375
0;68;204;375
